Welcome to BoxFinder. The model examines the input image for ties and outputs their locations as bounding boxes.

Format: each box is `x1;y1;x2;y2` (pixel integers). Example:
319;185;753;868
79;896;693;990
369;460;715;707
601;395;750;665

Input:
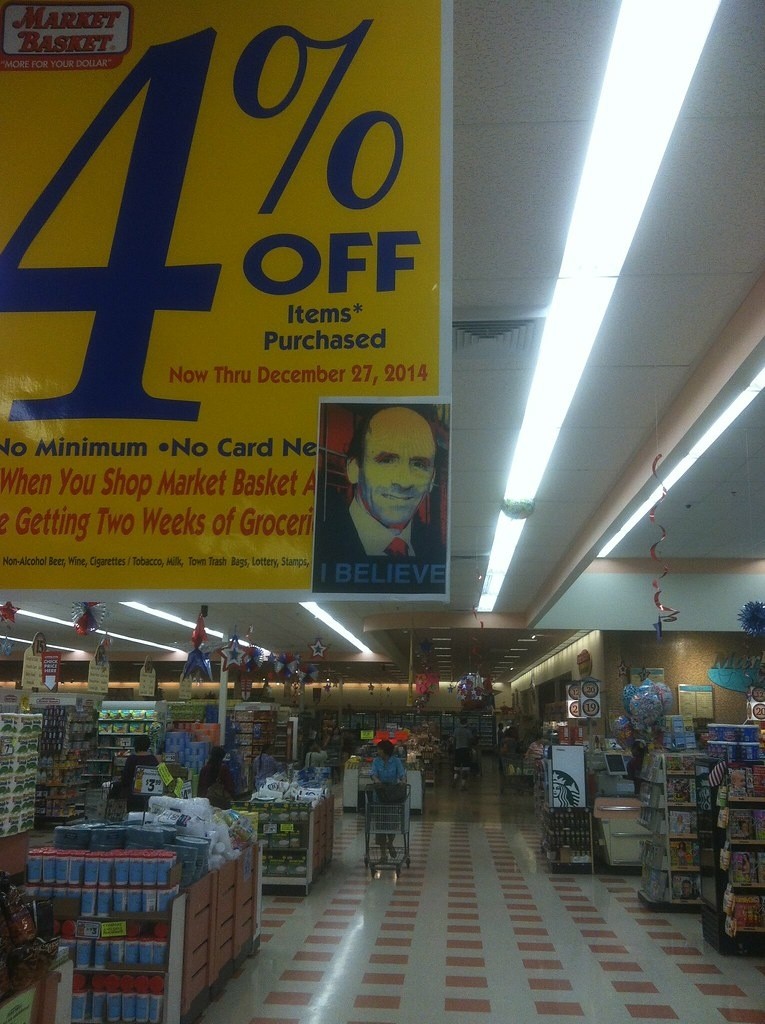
383;536;409;558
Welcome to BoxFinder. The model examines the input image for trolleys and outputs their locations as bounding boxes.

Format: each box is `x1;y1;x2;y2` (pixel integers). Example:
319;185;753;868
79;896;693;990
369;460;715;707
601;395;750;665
364;784;411;879
498;752;534;797
101;780;130;823
446;743;484;787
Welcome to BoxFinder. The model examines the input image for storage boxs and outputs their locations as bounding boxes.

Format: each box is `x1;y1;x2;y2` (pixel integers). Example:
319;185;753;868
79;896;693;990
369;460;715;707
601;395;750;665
261;800;312;896
0;713;44;838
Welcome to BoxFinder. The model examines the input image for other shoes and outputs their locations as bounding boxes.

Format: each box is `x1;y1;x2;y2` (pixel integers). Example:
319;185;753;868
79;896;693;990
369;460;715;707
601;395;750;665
388;845;396;858
380;855;388;864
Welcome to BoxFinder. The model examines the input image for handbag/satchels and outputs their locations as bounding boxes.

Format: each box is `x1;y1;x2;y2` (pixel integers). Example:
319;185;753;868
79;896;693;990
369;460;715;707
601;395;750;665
384;782;407;803
206;778;233;809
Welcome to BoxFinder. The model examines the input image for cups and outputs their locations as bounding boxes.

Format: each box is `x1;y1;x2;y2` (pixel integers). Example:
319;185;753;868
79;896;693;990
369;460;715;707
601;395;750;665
49;920;169;969
69;973;164;1023
28;847;180;917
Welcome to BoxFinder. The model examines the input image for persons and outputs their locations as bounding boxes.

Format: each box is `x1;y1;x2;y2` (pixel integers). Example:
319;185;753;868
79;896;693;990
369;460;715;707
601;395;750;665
675;842;687;866
450;717;476;791
370;740;406;858
197;746;233;810
730;769;748;796
740;821;750;839
681;880;694;900
497;723;505;750
674;813;687;834
324;404;447;594
252;742;276;777
121;735;160;813
323;726;344;783
524;733;544;762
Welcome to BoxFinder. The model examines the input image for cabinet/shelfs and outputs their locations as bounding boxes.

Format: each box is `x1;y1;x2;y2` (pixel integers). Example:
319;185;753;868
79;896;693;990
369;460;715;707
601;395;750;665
636;752;702;914
694;757;765;959
52;892;187;1024
336;713;497;752
35;701;301;831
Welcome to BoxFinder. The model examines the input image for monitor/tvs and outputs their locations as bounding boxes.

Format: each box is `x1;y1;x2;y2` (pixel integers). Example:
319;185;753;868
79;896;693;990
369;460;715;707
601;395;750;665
604;751;628;779
